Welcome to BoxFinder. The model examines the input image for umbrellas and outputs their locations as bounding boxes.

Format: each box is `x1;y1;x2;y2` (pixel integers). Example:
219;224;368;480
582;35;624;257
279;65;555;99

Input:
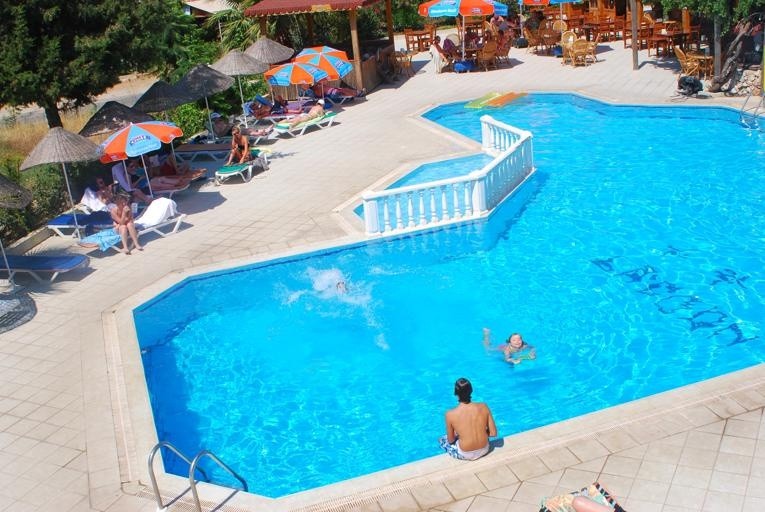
175;63;237;142
16;126;109;238
78;103;157;142
292;45;353;94
212;49;271;130
129;79;199;159
240;33;295;104
97;120;184;197
417;1;510;60
517;0;583;35
0;172;32;287
264;61;326;99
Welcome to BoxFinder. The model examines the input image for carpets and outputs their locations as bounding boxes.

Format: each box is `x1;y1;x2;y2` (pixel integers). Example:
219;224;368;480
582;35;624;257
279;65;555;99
0;289;38;334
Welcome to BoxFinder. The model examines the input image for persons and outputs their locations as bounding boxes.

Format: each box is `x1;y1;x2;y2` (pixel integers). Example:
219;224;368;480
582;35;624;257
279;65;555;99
124;160;189;189
483;324;536;367
280;100;329;128
226;125;250;166
95;178;162;203
211;113;273;135
336;282;348;297
309;84;360;99
252;102;305;117
433;8;546;72
109;194;144;252
572;495;622;511
139;153;191;176
432;379;501;462
